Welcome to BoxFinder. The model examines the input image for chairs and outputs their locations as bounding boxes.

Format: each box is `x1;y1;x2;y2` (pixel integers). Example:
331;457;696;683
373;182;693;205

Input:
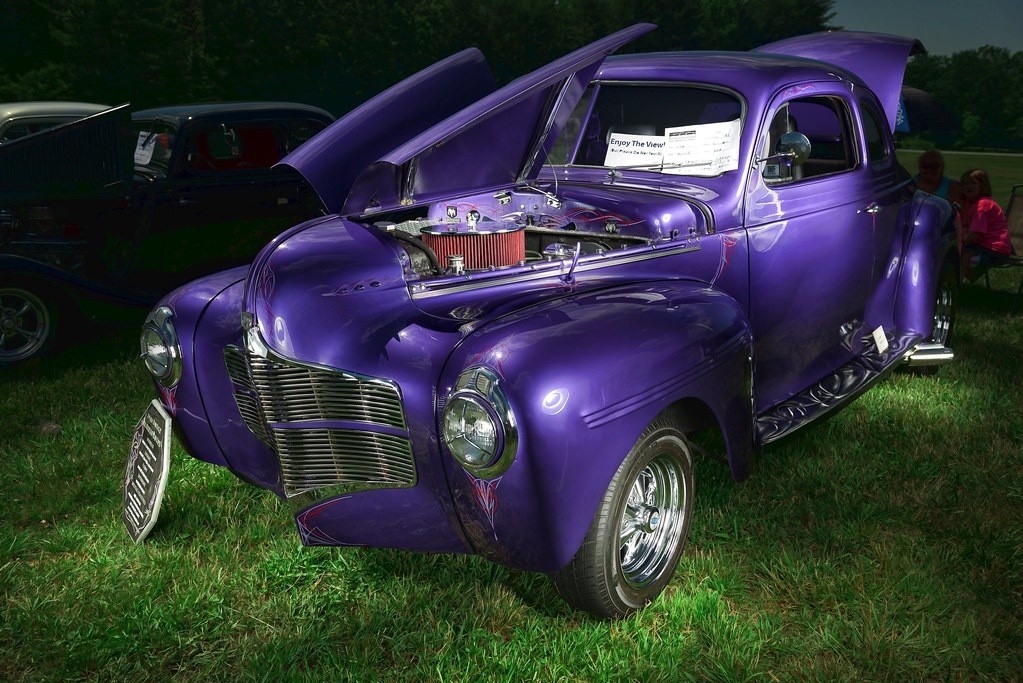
194;129;279;169
766;111;805;177
949;180;1023;313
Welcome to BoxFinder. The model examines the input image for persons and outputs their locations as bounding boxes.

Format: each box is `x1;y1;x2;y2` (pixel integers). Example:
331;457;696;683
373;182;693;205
913;151;961;202
957;169;1011;261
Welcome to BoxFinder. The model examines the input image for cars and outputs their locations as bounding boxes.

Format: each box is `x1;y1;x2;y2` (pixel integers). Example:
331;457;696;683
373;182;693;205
138;21;959;621
0;101;338;364
